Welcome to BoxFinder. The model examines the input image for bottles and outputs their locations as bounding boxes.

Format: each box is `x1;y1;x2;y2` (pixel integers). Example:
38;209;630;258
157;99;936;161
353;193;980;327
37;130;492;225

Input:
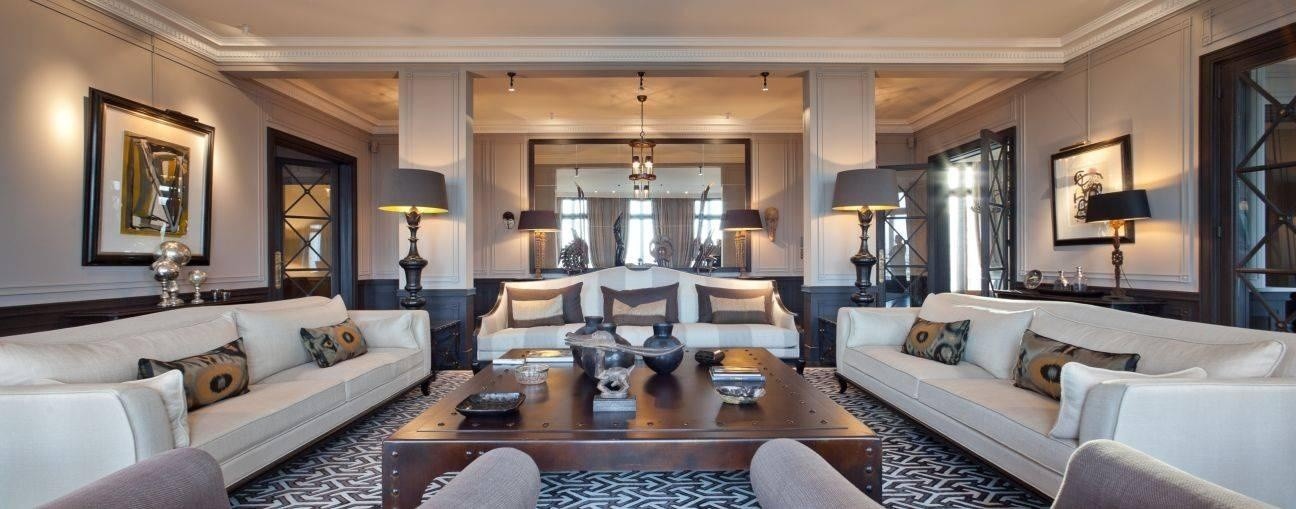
1054;268;1069;292
1073;267;1087;292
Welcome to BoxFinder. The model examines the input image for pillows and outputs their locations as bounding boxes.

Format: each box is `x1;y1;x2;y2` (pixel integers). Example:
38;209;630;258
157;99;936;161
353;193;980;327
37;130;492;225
0;310;238;386
1013;329;1141;403
137;337;250;412
355;312;419;350
506;282;583;328
300;317;368;368
846;310;918;348
1048;361;1209;439
32;370;190;448
233;295;348;385
918;293;1035;380
900;316;970;365
1028;306;1286;377
600;283;681;325
695;283;775;325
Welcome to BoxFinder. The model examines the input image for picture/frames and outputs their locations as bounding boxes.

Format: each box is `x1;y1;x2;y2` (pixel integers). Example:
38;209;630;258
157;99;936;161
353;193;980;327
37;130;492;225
1051;135;1135;245
81;88;216;267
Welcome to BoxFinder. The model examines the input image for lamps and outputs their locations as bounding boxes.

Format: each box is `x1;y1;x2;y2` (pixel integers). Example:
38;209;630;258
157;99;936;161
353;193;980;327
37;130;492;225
761;72;770;92
503;211;515;229
376;169;448;310
506;72;516;92
518;211;560;280
764;207;778;241
831;168;900;307
1085;189;1152;299
720;209;763;279
628;72;655;202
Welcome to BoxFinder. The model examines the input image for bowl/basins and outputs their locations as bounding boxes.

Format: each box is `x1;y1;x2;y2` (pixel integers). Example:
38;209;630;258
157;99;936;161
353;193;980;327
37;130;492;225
515;364;549;386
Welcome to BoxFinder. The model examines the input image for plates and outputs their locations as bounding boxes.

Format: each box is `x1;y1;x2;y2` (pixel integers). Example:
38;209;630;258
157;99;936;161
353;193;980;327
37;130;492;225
456;392;526;415
1023;270;1043;290
717;386;766;405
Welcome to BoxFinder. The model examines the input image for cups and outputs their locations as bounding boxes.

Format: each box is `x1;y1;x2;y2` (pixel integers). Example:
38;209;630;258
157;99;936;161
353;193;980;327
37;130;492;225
222;291;232;301
211;289;223;299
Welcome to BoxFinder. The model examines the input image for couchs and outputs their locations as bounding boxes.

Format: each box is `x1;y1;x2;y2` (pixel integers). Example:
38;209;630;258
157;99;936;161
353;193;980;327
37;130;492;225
30;446;541;508
471;266;806;375
836;293;1295;508
751;438;1277;509
1;295;436;509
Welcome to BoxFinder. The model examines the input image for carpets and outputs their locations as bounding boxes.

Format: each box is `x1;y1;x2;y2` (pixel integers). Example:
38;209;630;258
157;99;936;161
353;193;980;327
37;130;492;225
227;367;1056;507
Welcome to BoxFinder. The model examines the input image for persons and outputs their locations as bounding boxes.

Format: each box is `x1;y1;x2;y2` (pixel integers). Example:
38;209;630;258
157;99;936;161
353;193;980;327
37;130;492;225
889;235;906;276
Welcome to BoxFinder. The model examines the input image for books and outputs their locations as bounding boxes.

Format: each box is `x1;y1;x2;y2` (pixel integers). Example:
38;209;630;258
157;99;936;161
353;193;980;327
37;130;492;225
493;348;575;365
709;365;766;383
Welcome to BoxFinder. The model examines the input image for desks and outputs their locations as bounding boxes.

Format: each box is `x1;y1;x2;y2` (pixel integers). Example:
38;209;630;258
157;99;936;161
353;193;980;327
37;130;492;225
430;320;461;369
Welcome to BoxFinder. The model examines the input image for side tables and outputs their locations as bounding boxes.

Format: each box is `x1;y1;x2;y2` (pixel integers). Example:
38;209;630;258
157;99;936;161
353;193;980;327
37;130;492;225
819;314;837;364
